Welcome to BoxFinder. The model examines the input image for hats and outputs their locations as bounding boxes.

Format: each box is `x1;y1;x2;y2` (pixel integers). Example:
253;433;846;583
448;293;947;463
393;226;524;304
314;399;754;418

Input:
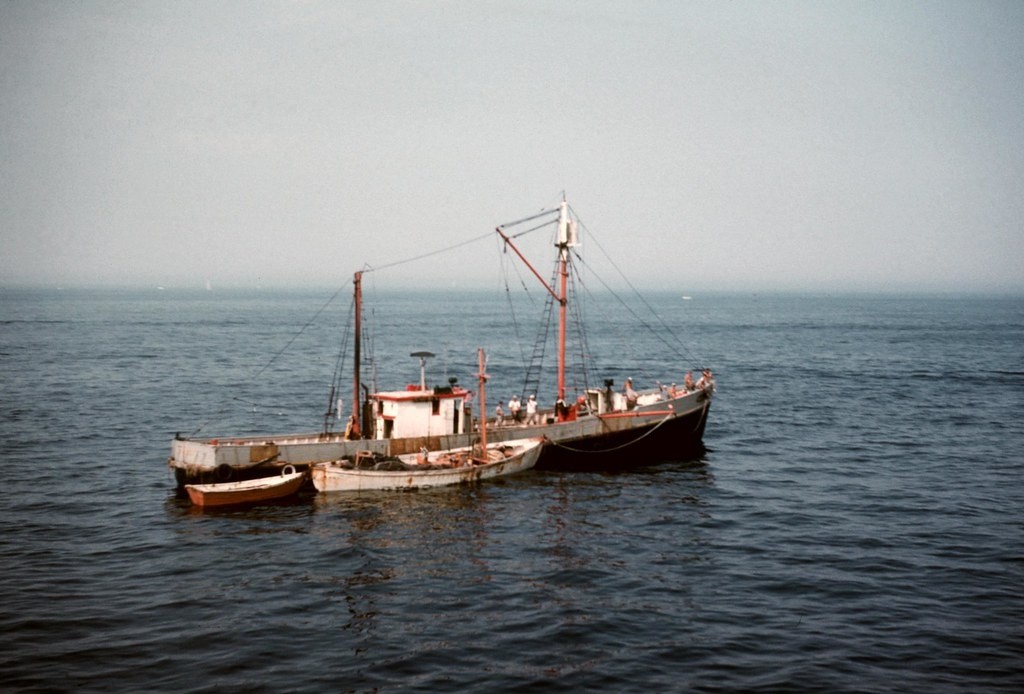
662;385;667;387
672;383;676;386
513;395;517;398
530;395;535;398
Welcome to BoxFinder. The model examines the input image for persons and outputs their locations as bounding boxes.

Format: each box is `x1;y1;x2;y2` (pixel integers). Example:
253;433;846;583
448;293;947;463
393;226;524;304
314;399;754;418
685;368;717;392
660;383;677;400
526;394;538;425
508;395;521;424
624;377;632;388
496;401;504;423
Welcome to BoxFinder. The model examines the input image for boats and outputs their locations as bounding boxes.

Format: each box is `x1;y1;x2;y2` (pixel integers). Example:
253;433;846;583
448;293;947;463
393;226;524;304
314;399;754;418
169;187;715;467
313;437;546;493
184;467;306;510
